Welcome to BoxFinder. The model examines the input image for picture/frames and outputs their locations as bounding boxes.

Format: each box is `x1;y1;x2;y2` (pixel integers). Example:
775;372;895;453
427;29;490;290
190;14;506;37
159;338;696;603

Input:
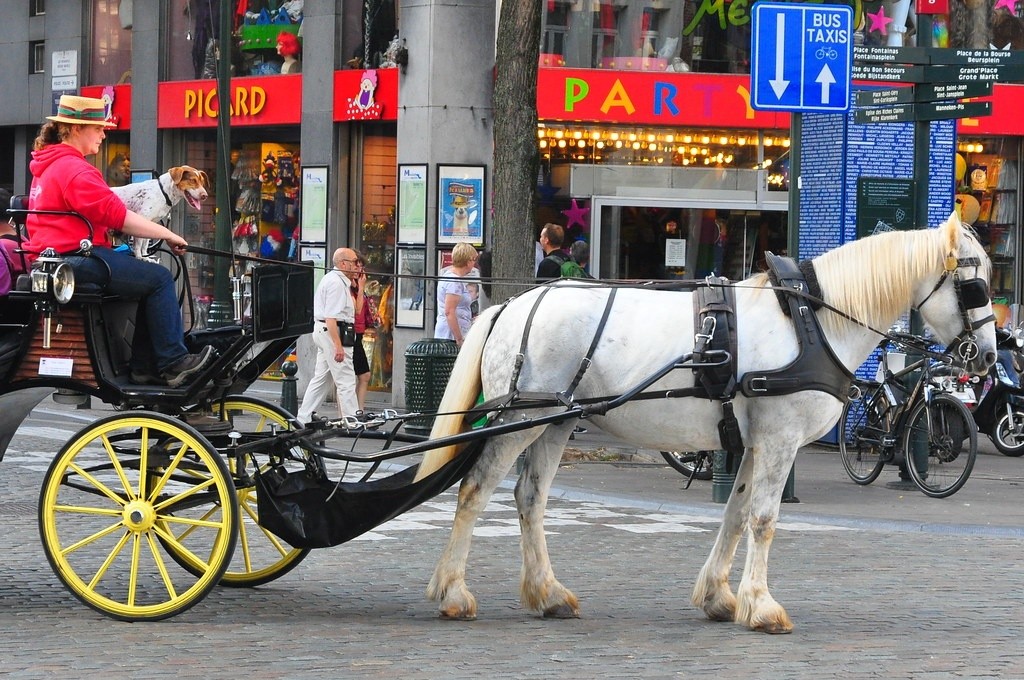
395;246;427;328
434;246;487;332
130;170;154;184
301;164;329;244
299;245;327;322
435;163;486;247
397;162;428;245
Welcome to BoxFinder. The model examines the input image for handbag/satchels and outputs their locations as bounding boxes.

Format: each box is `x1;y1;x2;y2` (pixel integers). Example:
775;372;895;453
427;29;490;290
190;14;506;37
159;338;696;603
340;323;355;346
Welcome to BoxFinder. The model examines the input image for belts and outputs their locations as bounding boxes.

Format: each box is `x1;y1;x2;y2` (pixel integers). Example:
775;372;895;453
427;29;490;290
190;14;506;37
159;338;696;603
319;320;344;326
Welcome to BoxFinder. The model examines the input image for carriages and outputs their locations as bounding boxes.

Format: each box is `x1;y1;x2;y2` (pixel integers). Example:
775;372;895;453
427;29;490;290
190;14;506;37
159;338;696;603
0;195;1001;637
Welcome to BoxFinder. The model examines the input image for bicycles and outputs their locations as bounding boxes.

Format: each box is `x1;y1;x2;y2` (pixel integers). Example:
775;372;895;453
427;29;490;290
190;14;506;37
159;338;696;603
836;332;978;499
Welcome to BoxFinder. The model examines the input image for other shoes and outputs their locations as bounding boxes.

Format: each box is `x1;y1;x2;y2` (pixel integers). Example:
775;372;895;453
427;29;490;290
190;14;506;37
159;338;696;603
573;426;587;434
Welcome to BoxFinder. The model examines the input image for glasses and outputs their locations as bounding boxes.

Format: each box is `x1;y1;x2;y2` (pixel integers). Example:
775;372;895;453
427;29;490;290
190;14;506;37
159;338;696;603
343;259;361;267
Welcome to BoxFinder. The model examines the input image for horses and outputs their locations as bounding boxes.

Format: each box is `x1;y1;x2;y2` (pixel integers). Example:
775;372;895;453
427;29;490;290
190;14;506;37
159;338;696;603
416;205;996;635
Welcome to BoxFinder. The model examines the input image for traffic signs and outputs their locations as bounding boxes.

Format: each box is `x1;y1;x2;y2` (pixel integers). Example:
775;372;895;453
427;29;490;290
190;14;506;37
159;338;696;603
751;0;854;115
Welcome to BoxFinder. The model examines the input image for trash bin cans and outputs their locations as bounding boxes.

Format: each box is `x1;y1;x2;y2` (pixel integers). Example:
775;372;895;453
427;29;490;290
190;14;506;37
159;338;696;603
403;337;461;435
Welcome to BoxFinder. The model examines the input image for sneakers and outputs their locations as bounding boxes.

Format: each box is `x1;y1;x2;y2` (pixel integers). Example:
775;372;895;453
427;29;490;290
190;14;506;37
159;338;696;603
130;369;166;386
162;344;215;388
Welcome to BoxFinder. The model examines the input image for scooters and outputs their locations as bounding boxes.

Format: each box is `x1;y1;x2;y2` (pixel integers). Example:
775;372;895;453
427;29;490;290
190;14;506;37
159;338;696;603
961;318;1024;456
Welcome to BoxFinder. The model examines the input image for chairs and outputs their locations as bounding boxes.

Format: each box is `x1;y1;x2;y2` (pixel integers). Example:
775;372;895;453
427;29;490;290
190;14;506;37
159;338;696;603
8;208;181;301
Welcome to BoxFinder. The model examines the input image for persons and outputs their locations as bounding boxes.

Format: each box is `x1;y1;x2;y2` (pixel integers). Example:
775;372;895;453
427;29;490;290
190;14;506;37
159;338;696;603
296;246;379;431
434;242;478;345
466;282;479;317
535;223;597;288
22;93;213;387
107;153;130;187
0;188;31;296
275;32;302;74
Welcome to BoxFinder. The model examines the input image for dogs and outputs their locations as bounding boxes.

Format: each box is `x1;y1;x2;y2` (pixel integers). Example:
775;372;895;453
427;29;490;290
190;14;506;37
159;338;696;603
108;165;211;263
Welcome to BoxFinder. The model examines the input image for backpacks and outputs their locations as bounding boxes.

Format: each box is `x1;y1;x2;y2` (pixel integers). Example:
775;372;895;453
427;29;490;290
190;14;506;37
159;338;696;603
545;252;589;278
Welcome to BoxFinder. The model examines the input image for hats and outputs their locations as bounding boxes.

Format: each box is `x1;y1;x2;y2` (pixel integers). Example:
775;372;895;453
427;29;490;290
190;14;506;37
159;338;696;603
46;95;117;127
